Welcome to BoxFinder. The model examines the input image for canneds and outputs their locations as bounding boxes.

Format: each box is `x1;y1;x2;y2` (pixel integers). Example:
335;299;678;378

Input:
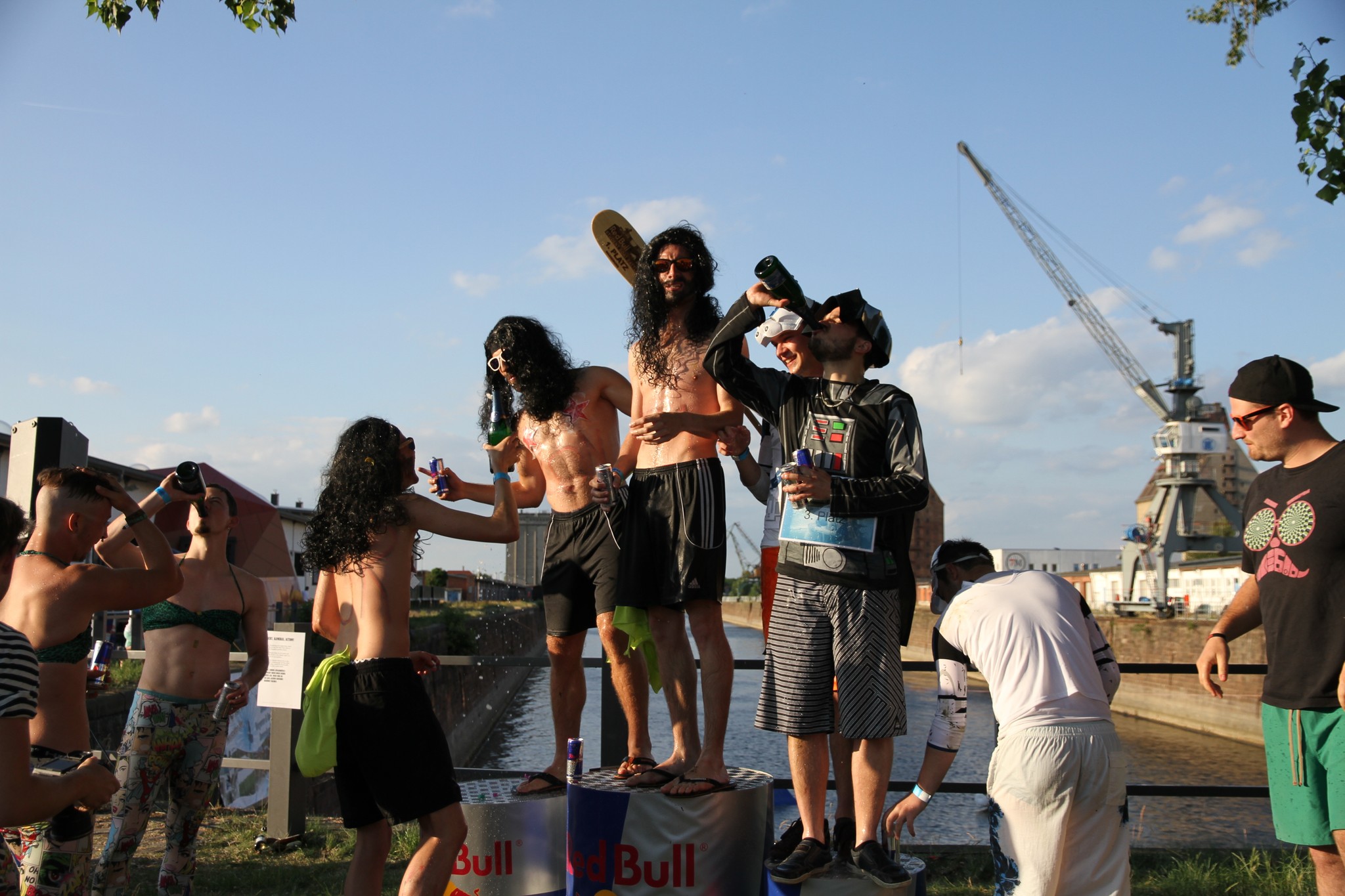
780;462;809;509
428;457;449;496
87;640;114;688
213;680;240;722
882;823;900;864
595;463;617;507
566;737;584;781
792;448;817;502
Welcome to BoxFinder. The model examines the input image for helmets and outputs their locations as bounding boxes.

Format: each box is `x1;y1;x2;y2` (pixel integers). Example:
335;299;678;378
820;286;892;368
752;292;823;347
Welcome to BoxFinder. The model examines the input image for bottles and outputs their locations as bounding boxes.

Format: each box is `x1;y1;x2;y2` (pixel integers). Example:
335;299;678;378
487;385;514;473
756;253;821;330
176;461;207;517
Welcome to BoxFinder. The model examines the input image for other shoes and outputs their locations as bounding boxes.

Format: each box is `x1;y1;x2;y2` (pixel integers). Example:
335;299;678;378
832;819;856;851
766;816;833;869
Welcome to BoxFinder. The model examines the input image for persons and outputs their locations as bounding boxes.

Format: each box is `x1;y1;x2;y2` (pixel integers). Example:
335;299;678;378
589;228;750;797
91;471;269;896
298;415;525;896
703;279;930;890
881;538;1131;896
419;316;660;798
0;467;185;896
1197;355;1345;896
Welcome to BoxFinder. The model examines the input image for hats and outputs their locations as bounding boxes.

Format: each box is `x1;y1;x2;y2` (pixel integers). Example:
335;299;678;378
1228;354;1338;421
928;540;990;616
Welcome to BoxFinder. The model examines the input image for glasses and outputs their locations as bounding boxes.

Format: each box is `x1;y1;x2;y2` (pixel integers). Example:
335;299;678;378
487;348;512;371
399;437;415;450
655;258;693;272
1229;406;1272;431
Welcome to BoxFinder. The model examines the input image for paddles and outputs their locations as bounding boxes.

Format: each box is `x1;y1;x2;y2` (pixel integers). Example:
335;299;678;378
592;209;762;434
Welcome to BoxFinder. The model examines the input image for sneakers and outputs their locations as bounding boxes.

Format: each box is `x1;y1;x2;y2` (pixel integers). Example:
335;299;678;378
769;837;833;883
845;843;912;887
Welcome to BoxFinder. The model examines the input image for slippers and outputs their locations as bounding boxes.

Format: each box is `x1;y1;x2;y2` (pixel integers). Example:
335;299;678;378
615;755;657;779
624;764;678;790
512;772;567;795
662;773;737;798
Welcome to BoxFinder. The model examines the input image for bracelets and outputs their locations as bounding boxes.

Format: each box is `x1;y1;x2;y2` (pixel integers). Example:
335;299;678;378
492;471;511;485
731;446;749;461
612;467;624;486
125;509;148;527
1206;633;1230;644
155;486;172;505
912;784;934;804
